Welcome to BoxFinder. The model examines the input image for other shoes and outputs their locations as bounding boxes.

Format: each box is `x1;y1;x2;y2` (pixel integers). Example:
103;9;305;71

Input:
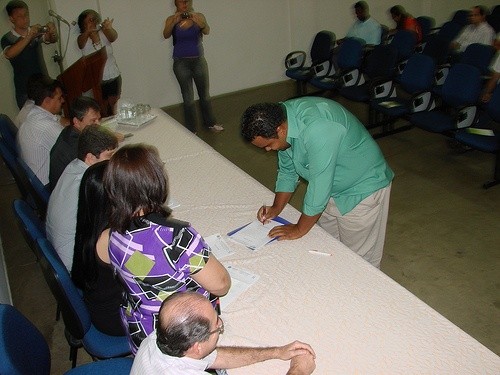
204;123;224;133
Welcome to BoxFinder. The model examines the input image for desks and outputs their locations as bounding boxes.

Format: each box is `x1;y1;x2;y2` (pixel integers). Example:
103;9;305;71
83;101;499;375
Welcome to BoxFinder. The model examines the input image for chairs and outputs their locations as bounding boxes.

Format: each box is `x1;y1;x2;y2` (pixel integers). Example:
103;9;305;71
0;113;196;375
284;4;500;189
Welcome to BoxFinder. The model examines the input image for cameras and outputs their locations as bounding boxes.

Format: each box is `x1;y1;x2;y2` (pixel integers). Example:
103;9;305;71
40;26;50;33
182;13;190;18
96;24;102;31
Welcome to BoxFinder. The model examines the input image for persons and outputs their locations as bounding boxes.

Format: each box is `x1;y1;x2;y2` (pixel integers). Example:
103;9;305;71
346;1;500;154
48;95;125;194
68;159;130;337
162;0;225;135
76;8;123;117
96;141;232;373
129;289;316;375
12;71;52;127
44;123;120;278
239;95;396;270
0;0;59;112
15;77;72;193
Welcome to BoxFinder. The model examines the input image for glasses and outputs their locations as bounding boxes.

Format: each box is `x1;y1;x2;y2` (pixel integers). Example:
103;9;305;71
84;16;101;22
209;316;224;334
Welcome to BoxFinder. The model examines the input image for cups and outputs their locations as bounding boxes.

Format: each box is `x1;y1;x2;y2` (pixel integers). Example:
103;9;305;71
119;103;151;124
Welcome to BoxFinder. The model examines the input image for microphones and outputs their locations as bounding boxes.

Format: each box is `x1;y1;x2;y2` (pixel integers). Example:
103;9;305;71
48;9;68;24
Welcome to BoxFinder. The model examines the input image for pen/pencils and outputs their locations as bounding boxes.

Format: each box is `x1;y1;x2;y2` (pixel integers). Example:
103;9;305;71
262;201;266;225
308;249;332;256
